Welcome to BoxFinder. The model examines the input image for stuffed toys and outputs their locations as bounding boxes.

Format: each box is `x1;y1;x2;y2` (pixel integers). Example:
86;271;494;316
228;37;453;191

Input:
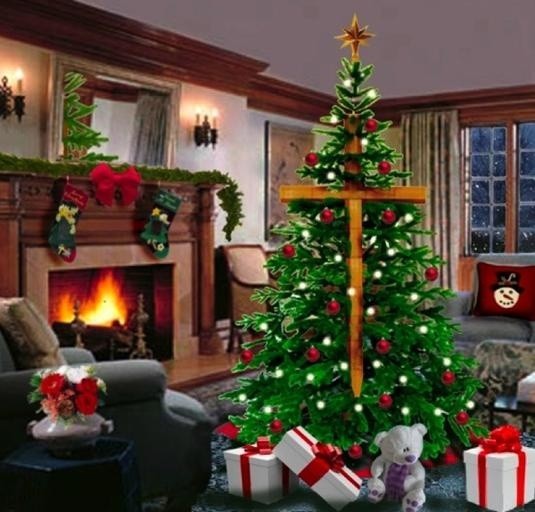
366;420;430;511
